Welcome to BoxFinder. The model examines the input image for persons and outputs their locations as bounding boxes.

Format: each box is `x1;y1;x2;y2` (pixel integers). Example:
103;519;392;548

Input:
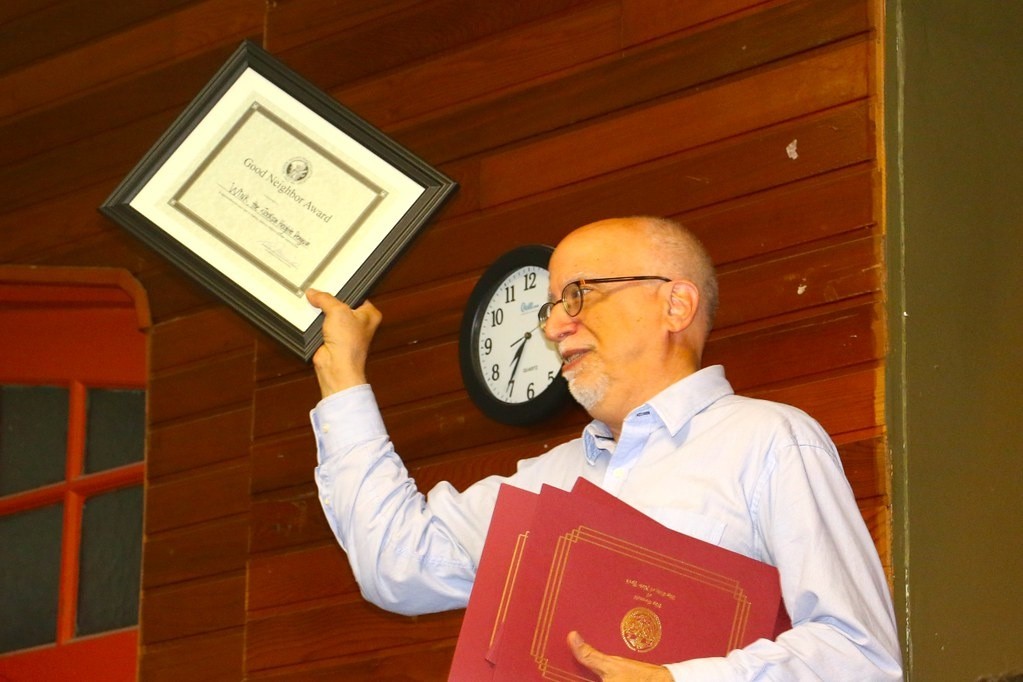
307;216;905;682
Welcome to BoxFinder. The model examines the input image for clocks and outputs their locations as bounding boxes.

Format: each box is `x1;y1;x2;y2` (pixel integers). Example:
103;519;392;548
459;244;581;426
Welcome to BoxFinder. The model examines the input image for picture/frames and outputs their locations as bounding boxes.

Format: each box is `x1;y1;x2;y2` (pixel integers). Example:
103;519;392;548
97;37;458;363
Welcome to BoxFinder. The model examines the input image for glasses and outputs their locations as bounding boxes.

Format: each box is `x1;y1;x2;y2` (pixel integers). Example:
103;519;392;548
538;276;671;333
